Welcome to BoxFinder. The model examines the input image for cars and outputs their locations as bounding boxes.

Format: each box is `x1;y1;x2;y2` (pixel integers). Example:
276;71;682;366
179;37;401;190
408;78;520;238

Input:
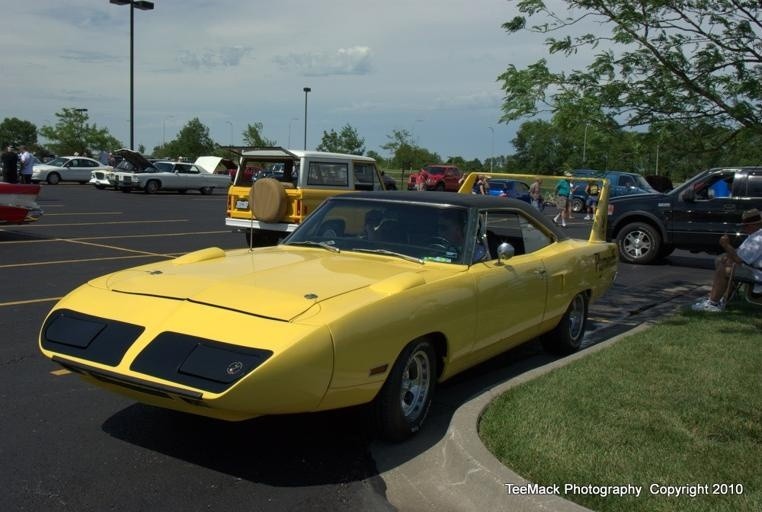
1;148;232;196
0;182;42;226
468;178;544;213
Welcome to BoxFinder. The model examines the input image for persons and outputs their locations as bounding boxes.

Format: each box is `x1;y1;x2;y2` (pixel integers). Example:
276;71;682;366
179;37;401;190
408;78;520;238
19;145;34;184
691;208;761;312
709;179;730;197
561;179;580;219
414;168;428;191
0;144;18;184
108;153;116;169
584;178;599;220
458;173;491;195
551;173;572;229
528;177;543;213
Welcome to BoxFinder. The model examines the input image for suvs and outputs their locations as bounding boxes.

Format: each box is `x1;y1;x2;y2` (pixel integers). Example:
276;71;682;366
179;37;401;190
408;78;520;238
224;146;398;248
557;170;664;214
597;166;762;264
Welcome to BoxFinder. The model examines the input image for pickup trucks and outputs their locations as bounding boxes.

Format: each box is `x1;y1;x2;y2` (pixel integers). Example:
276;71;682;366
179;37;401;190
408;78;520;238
407;165;466;192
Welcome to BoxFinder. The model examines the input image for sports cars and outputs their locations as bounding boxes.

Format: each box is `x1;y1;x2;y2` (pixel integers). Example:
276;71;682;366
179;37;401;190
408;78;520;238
39;172;620;439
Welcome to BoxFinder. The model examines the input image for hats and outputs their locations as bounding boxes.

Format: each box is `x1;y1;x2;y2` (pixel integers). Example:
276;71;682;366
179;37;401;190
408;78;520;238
736;209;762;226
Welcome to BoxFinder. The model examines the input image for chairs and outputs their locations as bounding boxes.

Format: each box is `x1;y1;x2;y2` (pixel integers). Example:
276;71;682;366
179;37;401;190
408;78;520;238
720;262;762;309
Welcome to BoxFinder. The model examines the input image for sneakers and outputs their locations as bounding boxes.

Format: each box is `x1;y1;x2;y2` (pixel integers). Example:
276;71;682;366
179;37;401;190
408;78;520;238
691;300;722;314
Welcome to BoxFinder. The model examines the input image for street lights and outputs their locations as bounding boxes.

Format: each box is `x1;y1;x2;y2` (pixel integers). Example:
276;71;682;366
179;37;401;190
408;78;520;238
109;1;154;151
225;120;233;144
304;88;311;151
126;112;738;177
161;115;172;144
289;117;300;147
75;108;88;147
